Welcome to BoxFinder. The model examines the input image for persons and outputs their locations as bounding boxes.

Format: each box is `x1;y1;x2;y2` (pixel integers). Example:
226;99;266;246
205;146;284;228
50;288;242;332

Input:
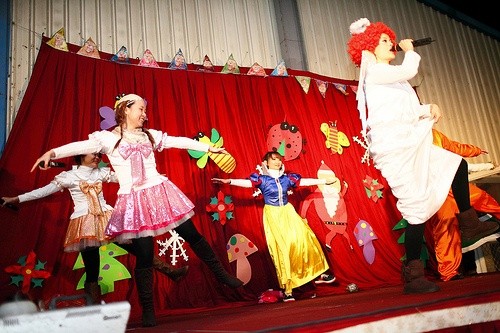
30;93;243;328
0;150;190;307
210;152;337;303
428;128;500;282
347;17;500;293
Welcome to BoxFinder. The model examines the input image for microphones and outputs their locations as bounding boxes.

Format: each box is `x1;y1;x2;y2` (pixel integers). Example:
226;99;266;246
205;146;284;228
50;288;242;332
40;161;66;168
392;37;436;51
0;198;19;211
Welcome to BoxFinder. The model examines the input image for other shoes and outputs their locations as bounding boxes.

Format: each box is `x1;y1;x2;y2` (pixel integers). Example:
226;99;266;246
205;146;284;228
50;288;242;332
283;293;295;302
315;274;335;284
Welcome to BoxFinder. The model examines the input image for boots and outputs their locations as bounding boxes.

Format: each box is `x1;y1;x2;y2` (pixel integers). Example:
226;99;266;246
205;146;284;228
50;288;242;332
455;206;500;240
190;237;244;288
134;267;154;327
402;259;440;293
152;256;189;282
85;282;101;306
